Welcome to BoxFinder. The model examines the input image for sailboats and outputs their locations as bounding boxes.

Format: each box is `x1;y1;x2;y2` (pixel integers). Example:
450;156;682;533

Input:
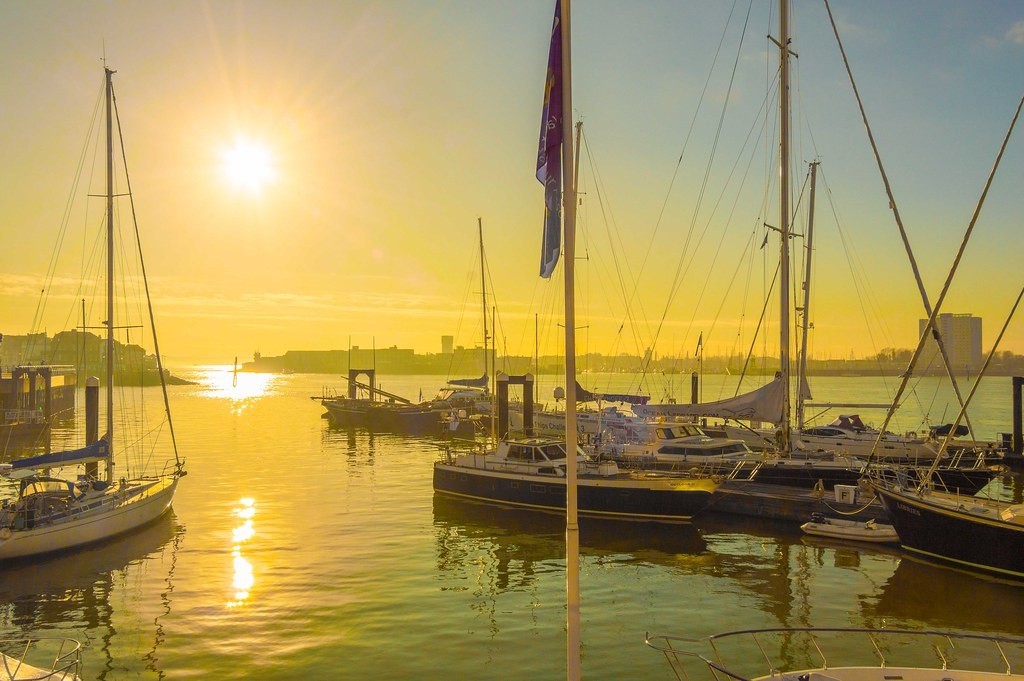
309;5;1022;587
0;66;186;559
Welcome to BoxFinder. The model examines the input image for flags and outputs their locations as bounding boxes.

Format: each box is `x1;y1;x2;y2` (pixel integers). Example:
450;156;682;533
534;2;562;282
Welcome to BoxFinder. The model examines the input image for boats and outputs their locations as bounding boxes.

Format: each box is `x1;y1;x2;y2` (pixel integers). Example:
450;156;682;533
640;628;1022;679
